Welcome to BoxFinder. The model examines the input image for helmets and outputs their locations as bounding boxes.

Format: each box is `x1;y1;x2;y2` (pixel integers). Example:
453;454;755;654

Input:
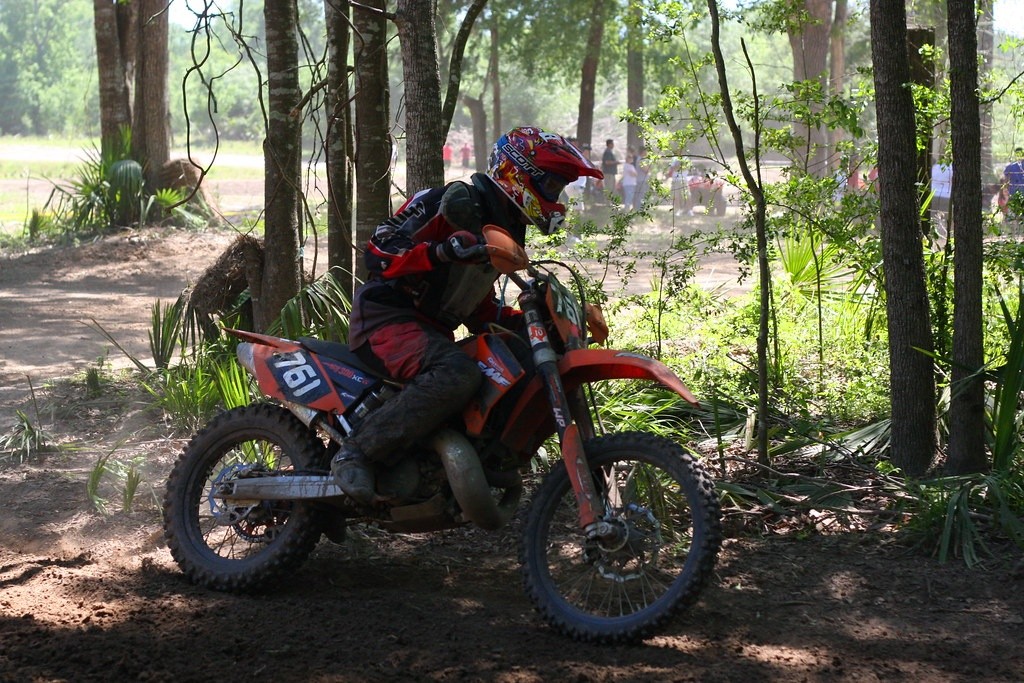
485;125;603;236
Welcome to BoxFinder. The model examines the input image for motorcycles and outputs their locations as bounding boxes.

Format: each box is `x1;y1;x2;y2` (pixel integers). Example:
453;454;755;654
161;221;723;646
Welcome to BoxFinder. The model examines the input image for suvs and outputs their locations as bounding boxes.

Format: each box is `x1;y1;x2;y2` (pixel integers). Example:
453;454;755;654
669;158;726;217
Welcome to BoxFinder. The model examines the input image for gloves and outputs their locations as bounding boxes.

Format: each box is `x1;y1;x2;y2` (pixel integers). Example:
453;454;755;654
445;230;488;263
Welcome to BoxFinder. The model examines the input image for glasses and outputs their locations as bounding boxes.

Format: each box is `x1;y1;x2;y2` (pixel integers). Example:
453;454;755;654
539;173;569;202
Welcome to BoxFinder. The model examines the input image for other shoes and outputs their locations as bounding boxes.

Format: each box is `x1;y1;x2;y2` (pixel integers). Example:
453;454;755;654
332;442;375;499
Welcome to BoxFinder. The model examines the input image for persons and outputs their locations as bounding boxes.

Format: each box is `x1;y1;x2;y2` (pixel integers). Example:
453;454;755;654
664;148;726;216
931;156;953;213
622;145;650;209
460;143;472;170
556;138;607;240
322;124;605;543
444;142;453;169
999;147;1024;199
602;138;620;206
832;165;880;232
